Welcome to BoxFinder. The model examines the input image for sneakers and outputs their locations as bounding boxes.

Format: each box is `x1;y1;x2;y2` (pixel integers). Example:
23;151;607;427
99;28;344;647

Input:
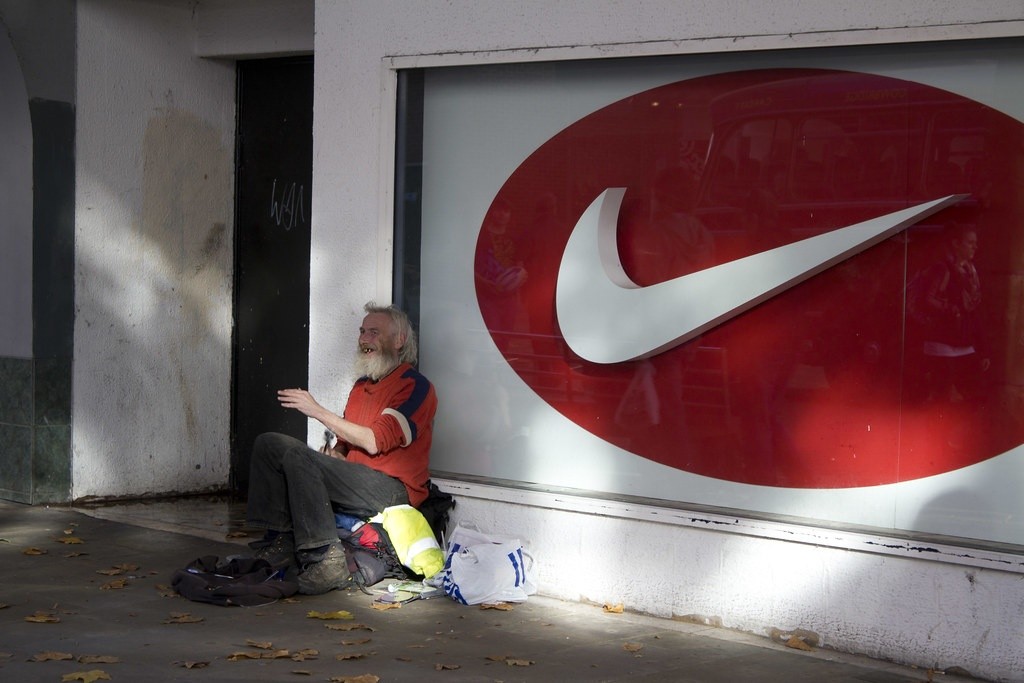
250;530;296;570
295;540;351;594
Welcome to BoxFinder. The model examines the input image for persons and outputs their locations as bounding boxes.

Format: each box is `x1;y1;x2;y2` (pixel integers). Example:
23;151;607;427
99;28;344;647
241;301;438;595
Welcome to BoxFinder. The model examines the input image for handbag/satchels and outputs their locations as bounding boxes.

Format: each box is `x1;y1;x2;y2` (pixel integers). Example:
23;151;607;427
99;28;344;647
172;556;299;607
350;481;533;606
